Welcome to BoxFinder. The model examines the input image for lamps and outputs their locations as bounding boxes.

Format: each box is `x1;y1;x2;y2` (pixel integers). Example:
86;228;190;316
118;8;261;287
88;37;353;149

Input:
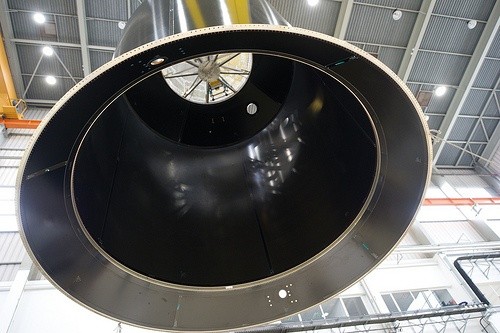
467;20;477;29
391;8;403;21
